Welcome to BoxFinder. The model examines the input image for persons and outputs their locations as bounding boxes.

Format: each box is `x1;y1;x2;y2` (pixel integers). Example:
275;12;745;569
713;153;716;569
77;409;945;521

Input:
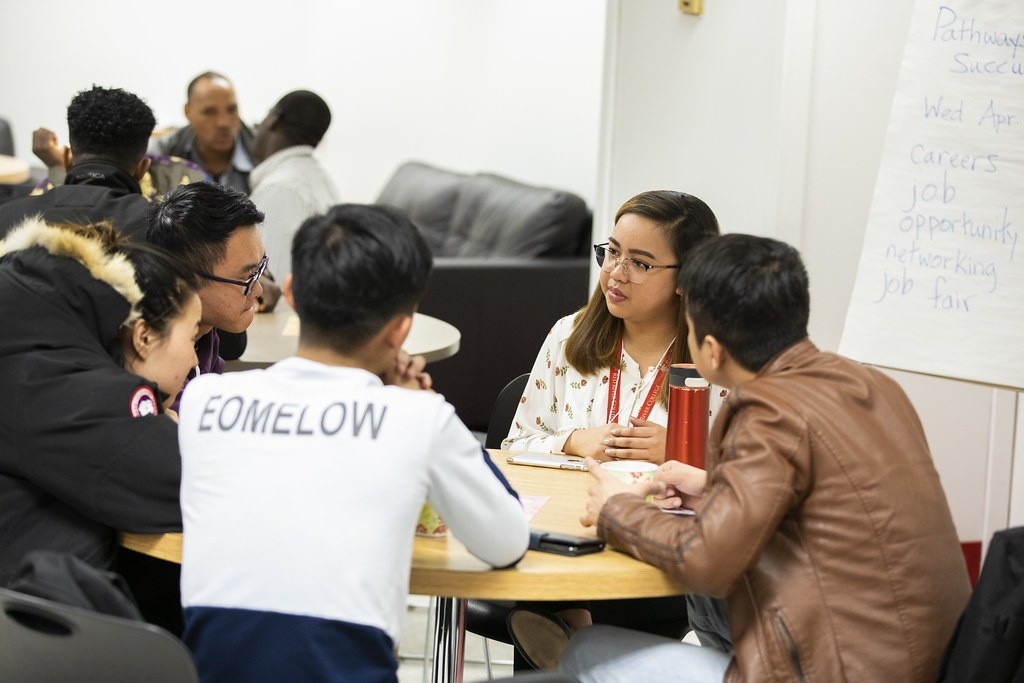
0;213;204;589
178;201;530;683
113;181;268;638
0;69;336;369
500;190;732;467
556;233;975;683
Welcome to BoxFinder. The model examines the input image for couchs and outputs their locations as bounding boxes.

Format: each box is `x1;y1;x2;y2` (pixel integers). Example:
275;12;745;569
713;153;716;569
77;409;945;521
373;161;595;432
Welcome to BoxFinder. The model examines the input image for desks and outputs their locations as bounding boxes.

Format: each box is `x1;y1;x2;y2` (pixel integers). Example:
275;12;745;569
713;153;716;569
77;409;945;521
121;448;685;682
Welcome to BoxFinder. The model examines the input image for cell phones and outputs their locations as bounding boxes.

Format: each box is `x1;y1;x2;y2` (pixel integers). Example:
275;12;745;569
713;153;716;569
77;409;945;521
530;528;606;558
506;451;603;472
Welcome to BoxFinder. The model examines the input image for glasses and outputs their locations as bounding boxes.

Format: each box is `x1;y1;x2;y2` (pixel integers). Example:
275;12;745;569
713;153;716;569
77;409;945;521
594;243;685;284
192;253;269;295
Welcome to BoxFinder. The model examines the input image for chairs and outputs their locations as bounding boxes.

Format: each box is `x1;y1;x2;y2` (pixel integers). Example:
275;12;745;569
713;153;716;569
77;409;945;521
940;527;1024;683
421;372;532;683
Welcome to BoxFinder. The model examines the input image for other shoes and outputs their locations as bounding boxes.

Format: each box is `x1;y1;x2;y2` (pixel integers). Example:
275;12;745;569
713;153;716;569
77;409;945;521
506;606;573;672
